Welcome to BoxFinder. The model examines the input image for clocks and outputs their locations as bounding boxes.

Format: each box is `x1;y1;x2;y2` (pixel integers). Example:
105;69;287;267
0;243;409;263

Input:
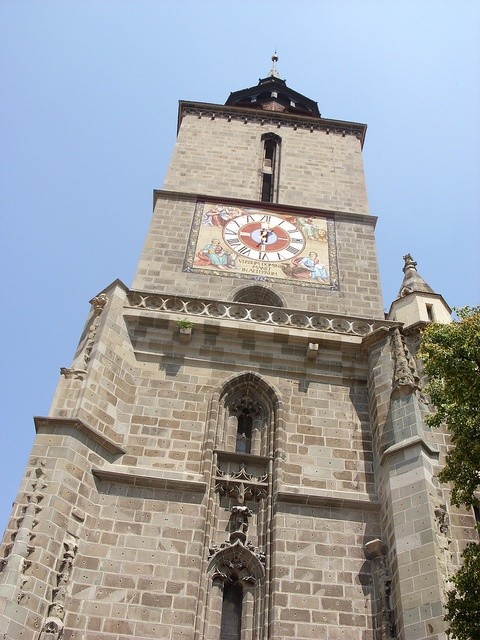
195;203;329;284
